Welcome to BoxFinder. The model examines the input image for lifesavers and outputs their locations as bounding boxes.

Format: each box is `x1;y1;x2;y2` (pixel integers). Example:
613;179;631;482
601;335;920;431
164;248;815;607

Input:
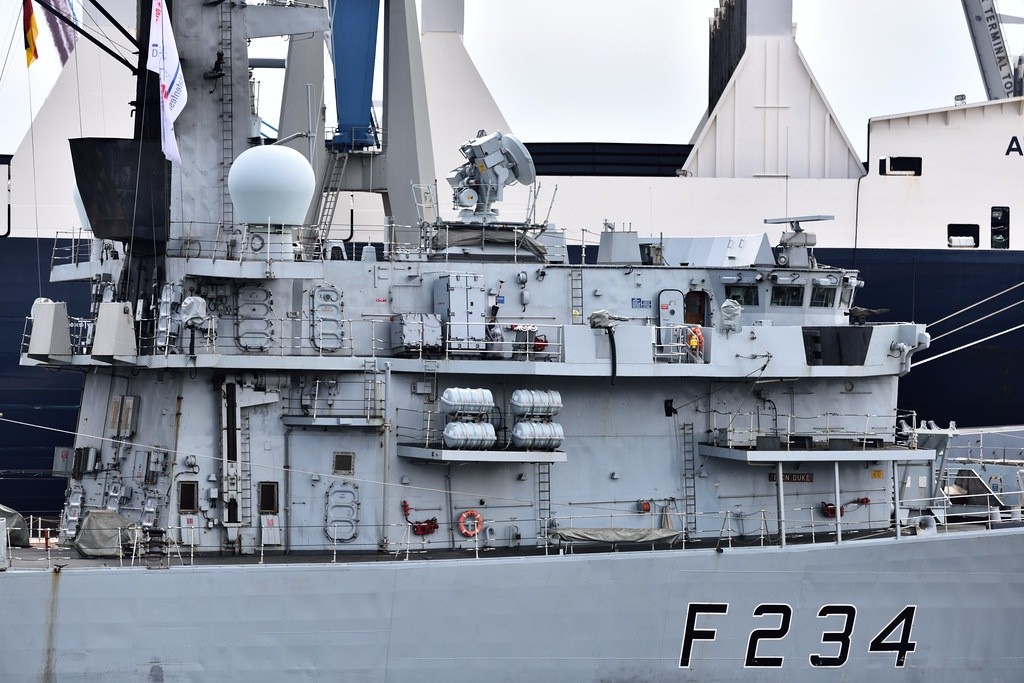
687;327;704;351
458;509;483;536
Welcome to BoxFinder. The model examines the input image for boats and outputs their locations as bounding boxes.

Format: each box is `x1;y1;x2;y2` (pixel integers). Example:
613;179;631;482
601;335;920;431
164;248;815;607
0;0;1024;683
